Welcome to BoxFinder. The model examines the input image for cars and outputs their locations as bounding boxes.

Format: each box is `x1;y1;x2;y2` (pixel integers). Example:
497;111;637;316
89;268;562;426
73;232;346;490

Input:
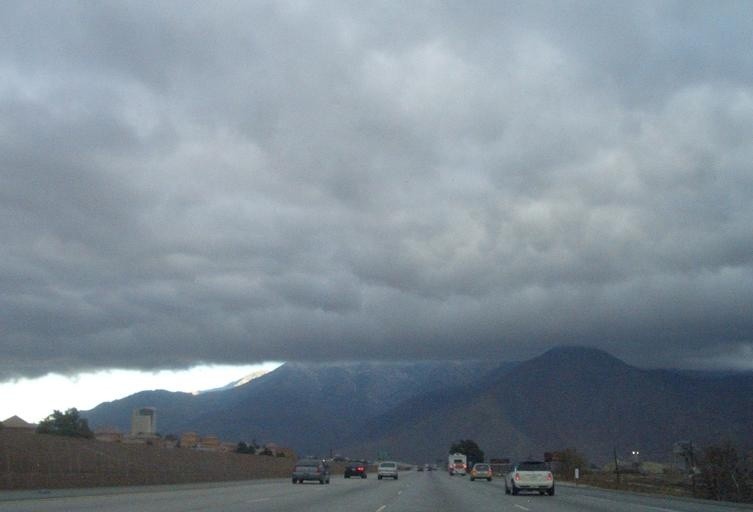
291;460;330;485
504;460;554;496
448;463;467;476
344;461;367;479
470;463;493;481
377;461;398;480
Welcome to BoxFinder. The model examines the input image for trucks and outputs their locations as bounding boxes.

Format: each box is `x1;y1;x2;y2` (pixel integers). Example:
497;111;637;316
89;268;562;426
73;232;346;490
448;452;467;465
